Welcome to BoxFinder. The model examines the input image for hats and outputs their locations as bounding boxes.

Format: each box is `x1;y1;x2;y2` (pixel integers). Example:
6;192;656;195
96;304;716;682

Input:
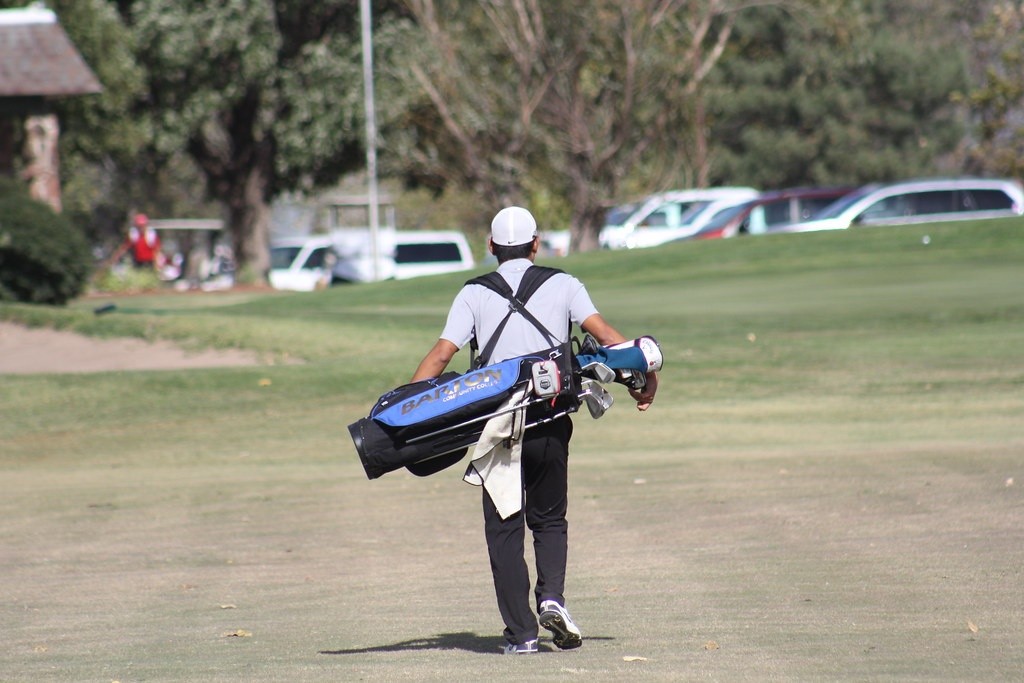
134;214;147;225
492;206;536;246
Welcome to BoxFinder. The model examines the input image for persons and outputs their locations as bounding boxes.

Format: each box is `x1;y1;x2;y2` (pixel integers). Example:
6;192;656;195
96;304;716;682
411;206;658;655
113;214;159;271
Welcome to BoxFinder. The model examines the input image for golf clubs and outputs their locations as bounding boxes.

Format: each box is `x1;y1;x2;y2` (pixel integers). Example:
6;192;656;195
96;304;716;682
580;361;617;420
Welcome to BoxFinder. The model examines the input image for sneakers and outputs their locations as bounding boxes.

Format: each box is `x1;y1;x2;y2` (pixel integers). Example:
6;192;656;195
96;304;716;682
539;600;582;649
496;637;539;655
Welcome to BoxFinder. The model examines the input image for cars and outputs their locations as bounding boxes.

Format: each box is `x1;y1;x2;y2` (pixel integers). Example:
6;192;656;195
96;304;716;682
541;181;1024;256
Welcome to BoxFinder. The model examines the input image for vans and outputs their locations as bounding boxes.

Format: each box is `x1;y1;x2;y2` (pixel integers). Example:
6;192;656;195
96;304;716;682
268;230;477;292
